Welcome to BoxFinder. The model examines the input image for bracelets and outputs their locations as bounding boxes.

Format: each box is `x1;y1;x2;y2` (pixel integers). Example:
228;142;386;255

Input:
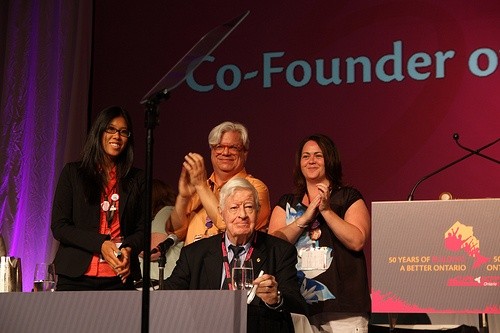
297;223;308;228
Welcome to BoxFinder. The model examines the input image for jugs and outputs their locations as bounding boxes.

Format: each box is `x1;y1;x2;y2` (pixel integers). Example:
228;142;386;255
0;256;22;292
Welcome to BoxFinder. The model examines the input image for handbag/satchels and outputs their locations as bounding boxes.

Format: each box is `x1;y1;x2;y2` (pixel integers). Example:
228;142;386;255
0;256;22;293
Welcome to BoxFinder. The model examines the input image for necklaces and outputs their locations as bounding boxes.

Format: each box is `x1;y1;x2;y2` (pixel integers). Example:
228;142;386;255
102;181;118;211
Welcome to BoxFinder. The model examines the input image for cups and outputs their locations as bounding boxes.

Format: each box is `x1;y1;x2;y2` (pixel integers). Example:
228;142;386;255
33;262;56;292
231;258;254;298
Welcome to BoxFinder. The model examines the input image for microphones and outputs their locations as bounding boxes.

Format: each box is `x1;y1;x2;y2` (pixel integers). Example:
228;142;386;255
152;234;178;253
453;133;500;166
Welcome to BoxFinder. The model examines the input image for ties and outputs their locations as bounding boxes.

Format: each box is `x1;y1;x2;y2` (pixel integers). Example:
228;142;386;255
222;245;245;290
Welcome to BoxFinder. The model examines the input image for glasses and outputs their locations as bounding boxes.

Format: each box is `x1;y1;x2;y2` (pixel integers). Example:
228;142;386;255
104;126;131;137
212;144;244;154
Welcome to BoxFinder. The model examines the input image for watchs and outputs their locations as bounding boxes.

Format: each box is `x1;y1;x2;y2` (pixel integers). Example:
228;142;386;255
267;291;281;307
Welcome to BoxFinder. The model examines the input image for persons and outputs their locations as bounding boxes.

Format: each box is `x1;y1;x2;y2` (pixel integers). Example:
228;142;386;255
132;178;185;291
166;121;271;247
49;105;144;292
268;134;372;333
157;178;305;333
